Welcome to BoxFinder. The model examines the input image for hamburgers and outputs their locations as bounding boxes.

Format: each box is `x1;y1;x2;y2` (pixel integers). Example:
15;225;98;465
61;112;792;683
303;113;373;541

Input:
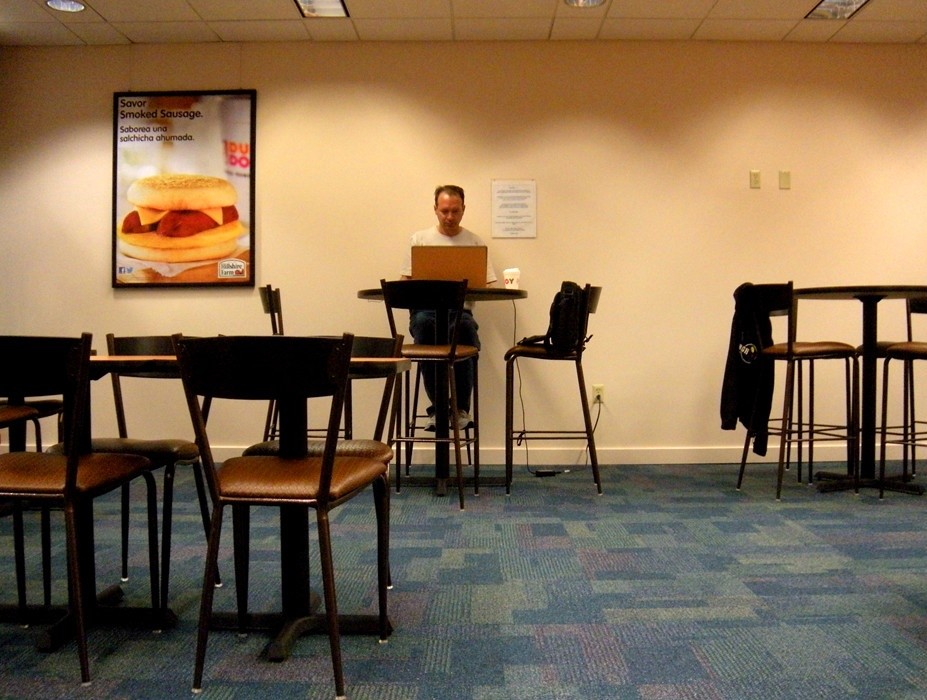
117;175;247;264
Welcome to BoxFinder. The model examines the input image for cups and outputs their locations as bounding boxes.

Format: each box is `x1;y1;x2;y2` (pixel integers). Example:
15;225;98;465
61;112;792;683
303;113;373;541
503;269;521;290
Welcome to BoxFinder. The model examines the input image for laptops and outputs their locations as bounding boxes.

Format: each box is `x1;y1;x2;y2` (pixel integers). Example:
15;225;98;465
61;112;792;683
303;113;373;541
412;246;488;288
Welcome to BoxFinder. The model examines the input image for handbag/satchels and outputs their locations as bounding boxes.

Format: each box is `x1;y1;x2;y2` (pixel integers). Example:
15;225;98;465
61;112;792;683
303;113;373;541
517;281;582;357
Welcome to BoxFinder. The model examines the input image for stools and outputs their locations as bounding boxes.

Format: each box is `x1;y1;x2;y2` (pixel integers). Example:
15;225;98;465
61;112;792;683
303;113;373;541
258;281;603;511
721;281;927;503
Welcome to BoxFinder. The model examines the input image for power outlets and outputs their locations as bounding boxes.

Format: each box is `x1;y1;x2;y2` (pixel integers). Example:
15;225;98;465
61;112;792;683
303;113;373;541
592;384;605;404
750;169;761;189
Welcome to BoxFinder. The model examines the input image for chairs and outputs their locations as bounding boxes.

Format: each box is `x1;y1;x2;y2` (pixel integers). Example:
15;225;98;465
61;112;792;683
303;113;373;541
0;333;404;694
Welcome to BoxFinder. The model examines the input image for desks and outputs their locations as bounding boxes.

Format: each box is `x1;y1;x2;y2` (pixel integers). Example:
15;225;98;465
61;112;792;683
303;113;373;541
0;356;177;660
794;285;927;497
357;285;528;496
153;356;412;665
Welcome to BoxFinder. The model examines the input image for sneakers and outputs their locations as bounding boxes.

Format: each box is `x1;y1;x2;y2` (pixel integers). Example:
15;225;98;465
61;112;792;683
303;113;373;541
424;415;436;431
449;408;472;430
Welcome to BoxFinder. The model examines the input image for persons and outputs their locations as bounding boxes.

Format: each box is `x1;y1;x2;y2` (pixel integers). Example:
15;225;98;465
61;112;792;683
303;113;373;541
398;185;497;433
